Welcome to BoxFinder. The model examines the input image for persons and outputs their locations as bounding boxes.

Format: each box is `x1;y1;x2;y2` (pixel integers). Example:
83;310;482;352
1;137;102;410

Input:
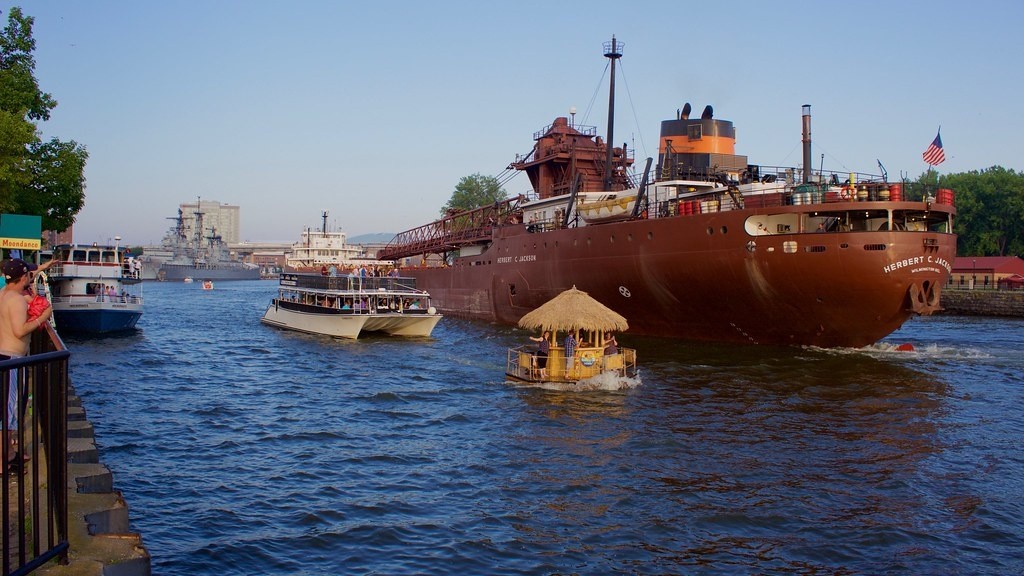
281;294;421;309
0;259;54;477
510;216;518;225
0;258;59;304
124;257;142;280
352;264;400;289
529;331;549;379
529;215;538;233
564;331;583;379
94;283;131;305
320;262;336;277
553;207;562;229
604;332;617;356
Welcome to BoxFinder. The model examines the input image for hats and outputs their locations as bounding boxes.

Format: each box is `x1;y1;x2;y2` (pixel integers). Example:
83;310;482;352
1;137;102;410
4;258;38;281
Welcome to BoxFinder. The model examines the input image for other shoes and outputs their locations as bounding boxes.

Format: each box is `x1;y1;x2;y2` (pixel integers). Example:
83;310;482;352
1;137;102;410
565;377;570;380
541;374;549;378
11;439;18;445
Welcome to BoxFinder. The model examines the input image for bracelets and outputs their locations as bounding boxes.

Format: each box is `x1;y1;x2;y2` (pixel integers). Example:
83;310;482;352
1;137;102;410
36;318;40;326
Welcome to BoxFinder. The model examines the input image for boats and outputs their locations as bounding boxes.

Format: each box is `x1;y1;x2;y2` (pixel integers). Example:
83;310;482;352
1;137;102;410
141;195;282;282
377;35;961;352
285;211;394;275
47;239;144;338
259;265;445;344
505;287;638;394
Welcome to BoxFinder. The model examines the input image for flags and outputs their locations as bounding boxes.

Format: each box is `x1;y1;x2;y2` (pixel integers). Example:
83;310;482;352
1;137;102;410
923;130;945;166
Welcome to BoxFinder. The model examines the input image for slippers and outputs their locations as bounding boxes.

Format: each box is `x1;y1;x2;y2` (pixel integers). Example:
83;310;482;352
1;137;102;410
0;452;31;478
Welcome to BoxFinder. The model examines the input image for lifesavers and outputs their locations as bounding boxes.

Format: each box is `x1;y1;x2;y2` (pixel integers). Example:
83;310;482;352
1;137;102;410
581;350;596;366
841;186;856;202
